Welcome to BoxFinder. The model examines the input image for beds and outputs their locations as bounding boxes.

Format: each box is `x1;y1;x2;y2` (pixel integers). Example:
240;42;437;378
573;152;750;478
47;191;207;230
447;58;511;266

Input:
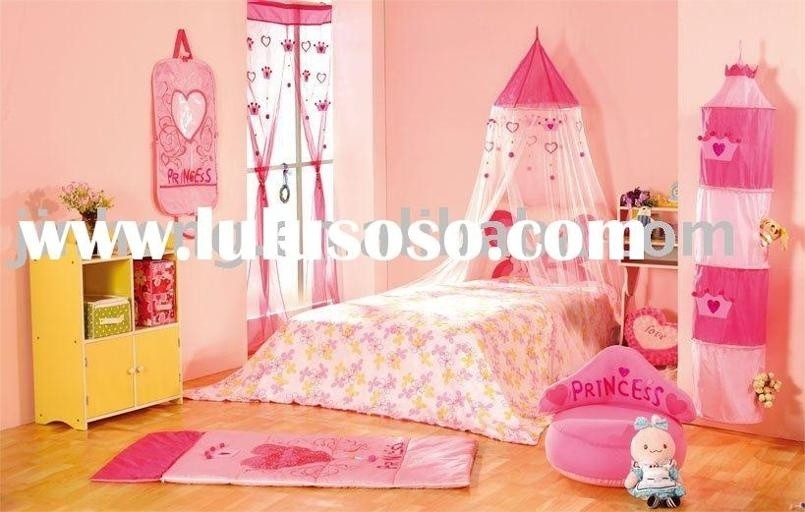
285;204;615;420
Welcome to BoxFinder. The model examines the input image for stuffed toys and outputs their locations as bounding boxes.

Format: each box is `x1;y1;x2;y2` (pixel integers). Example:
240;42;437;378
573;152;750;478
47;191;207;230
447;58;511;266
627;205;655;242
624;414;687;509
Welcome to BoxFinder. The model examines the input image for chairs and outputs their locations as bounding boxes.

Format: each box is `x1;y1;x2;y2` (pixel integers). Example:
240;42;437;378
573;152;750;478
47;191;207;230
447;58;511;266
537;342;700;488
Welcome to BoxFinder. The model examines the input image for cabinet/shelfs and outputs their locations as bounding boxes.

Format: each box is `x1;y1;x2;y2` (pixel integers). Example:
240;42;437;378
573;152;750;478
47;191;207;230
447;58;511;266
27;245;188;434
617;187;680;343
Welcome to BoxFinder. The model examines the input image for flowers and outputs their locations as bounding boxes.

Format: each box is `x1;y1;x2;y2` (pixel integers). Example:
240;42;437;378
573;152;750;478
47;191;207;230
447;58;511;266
751;369;784;411
59;180;118;215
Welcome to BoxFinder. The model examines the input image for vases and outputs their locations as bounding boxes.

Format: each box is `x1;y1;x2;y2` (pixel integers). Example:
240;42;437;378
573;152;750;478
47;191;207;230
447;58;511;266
76;207;106;257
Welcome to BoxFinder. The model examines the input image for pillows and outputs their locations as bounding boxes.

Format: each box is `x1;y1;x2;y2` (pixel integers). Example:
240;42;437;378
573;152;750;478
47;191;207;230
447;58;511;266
624;304;685;368
477;207;603;288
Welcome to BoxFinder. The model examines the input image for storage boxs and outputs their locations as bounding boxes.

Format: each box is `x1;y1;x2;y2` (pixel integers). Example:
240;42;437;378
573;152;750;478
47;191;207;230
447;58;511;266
135;257;176;293
133;292;176;327
83;291;133;339
